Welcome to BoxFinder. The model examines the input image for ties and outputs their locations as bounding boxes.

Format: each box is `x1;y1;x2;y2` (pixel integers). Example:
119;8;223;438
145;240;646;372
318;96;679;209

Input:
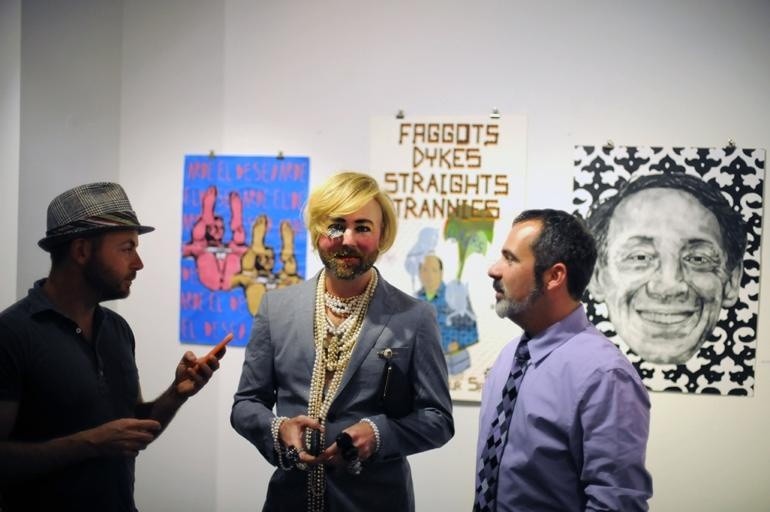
472;342;530;512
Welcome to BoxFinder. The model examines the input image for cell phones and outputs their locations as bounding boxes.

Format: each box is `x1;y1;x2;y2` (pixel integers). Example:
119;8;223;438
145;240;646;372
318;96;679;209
189;332;235;379
310;429;320;456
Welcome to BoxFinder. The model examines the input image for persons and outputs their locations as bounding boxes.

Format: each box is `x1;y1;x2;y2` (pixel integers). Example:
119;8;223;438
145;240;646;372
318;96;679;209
1;182;234;509
472;209;653;511
410;254;478;374
229;170;457;512
584;174;744;366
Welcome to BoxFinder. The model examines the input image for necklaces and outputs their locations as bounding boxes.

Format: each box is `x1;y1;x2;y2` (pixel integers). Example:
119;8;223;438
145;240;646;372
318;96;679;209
300;261;379;512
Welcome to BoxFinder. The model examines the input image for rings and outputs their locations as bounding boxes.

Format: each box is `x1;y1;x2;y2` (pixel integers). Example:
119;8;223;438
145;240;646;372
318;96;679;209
296;447;304;454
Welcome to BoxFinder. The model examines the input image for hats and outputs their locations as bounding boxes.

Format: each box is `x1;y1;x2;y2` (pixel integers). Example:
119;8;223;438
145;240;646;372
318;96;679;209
38;182;155;252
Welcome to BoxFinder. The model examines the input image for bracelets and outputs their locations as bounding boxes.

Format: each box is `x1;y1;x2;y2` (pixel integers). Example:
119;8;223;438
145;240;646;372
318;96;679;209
270;416;295;471
171;384;186;404
